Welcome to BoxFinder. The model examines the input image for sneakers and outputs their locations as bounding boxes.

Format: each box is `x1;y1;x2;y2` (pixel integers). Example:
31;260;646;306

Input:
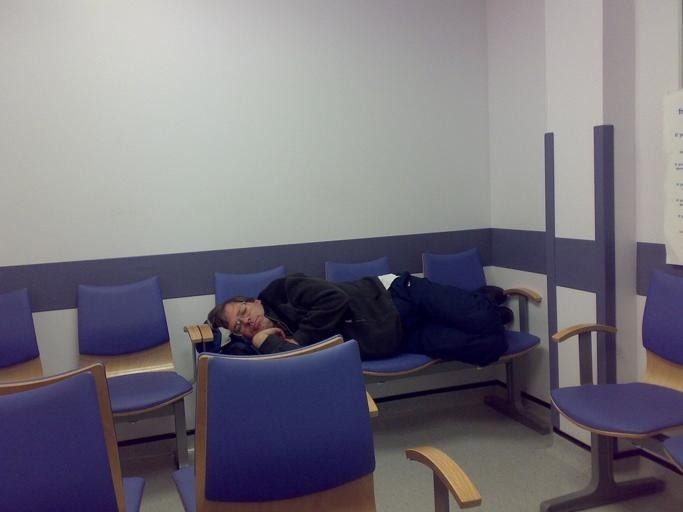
476;286;513;324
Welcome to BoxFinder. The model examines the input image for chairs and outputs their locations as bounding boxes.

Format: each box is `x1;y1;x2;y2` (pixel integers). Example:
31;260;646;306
172;335;483;512
3;362;145;510
77;276;193;470
215;251;540;468
530;261;681;511
3;289;42;379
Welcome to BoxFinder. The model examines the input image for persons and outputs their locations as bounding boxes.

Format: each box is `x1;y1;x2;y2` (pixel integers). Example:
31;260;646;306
208;275;513;367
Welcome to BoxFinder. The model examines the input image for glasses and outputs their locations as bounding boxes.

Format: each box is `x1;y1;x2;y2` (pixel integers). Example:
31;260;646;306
232;301;246;337
